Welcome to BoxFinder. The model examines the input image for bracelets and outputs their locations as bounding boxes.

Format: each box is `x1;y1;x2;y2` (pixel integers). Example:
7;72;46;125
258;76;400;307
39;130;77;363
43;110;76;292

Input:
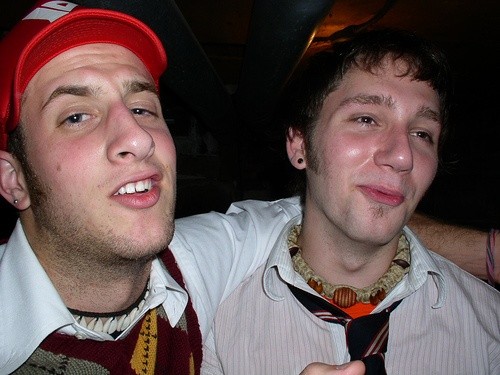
485;227;496;287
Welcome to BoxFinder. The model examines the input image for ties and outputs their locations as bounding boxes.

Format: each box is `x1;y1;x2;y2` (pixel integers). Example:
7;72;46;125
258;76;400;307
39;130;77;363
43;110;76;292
288;284;404;375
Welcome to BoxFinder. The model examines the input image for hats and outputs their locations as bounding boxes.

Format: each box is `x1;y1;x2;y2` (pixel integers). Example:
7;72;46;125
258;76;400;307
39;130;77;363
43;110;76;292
272;0;467;100
0;0;167;153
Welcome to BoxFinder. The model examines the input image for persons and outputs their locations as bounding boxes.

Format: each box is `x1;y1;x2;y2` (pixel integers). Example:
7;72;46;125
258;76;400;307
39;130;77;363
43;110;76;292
203;2;499;374
0;0;500;374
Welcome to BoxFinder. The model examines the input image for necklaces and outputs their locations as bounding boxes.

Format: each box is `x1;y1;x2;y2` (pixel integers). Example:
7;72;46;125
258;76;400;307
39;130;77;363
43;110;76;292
283;220;412;306
56;274;151;336
